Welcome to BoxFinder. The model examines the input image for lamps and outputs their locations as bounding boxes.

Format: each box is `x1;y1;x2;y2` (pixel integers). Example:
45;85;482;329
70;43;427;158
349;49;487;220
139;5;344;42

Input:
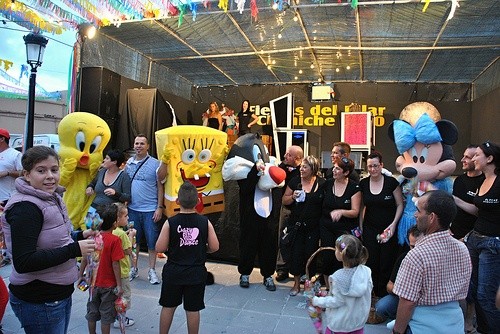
76;22;98;114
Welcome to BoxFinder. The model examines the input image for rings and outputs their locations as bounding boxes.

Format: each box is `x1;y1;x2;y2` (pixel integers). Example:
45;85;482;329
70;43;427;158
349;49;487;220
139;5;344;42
107;192;109;194
336;215;338;216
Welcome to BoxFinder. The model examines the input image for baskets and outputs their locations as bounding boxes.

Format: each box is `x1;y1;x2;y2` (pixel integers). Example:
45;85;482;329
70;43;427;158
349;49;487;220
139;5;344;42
364;290;384;324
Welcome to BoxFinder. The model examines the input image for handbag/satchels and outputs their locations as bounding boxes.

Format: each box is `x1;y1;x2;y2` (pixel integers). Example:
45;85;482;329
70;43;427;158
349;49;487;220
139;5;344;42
279;225;298;248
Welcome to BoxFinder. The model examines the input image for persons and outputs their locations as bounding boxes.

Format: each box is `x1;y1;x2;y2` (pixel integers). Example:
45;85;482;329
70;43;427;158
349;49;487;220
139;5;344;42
153;182;222;334
1;115;164;285
308;233;375;334
200;98;260;138
0;144;97;334
220;130;427;319
154;125;228;286
77;202;126;334
464;139;500;334
125;220;138;271
111;202;138;329
389;188;474;334
447;145;487;240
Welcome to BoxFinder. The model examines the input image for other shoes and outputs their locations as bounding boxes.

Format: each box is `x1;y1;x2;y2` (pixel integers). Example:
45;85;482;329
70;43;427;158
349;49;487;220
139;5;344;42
113;319;135;328
276;272;288;282
289;286;301;297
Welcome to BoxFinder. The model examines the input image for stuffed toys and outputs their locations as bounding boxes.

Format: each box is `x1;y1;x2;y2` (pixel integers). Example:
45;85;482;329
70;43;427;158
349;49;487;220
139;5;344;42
54;111;112;231
79;207;104;302
375;101;460;318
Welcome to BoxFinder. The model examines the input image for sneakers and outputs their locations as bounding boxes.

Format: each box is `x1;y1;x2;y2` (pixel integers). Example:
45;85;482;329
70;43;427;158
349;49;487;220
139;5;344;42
264;276;276;291
128;267;140;281
147;269;160;284
239;274;249;287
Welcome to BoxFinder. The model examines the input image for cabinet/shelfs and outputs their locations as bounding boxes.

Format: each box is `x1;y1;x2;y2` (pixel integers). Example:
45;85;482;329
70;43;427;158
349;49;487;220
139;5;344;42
78;67;122;152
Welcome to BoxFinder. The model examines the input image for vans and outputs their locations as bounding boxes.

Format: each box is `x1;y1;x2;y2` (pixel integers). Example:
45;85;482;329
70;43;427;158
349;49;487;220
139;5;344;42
11;133;60;156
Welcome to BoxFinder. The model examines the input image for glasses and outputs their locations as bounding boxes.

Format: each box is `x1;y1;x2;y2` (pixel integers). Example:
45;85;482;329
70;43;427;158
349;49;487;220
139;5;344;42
366;164;382;170
342;157;349;164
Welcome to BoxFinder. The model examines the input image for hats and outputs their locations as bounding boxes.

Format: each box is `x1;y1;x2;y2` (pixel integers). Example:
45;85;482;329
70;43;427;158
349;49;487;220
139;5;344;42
0;128;10;139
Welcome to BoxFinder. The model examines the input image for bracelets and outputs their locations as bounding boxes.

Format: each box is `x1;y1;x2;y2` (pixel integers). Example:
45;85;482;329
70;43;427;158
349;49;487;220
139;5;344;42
392;328;395;334
291;195;295;200
157;206;163;208
6;169;10;176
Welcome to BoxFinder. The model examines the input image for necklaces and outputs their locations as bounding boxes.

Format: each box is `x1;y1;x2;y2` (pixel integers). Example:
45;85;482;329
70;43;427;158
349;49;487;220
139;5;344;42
333;181;335;198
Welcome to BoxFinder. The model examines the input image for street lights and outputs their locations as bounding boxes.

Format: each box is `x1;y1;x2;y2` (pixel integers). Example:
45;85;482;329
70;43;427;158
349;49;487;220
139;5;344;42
22;28;48;155
